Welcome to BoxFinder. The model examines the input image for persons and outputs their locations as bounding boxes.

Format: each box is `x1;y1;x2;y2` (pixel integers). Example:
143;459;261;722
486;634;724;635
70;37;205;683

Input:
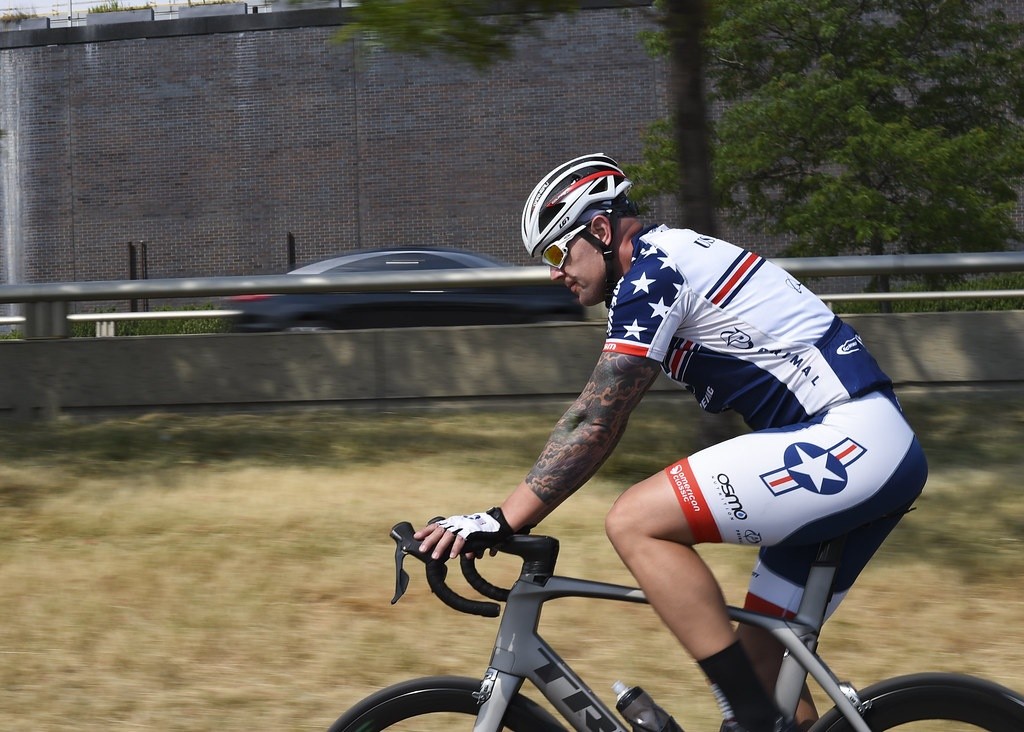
415;152;928;732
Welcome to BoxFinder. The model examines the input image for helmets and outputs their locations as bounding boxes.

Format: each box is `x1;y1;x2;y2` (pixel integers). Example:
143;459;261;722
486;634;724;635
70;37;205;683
522;153;635;259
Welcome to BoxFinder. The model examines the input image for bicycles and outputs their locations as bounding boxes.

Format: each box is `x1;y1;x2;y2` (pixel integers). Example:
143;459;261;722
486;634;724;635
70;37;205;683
327;517;1024;732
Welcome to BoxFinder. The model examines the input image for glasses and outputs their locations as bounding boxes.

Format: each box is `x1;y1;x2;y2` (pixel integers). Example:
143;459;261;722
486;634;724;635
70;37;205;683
542;211;611;270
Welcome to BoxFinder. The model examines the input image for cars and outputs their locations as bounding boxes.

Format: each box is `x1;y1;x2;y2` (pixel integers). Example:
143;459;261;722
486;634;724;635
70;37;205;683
222;246;587;332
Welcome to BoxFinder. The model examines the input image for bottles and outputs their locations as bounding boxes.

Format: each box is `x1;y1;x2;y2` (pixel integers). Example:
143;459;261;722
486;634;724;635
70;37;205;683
611;680;687;732
